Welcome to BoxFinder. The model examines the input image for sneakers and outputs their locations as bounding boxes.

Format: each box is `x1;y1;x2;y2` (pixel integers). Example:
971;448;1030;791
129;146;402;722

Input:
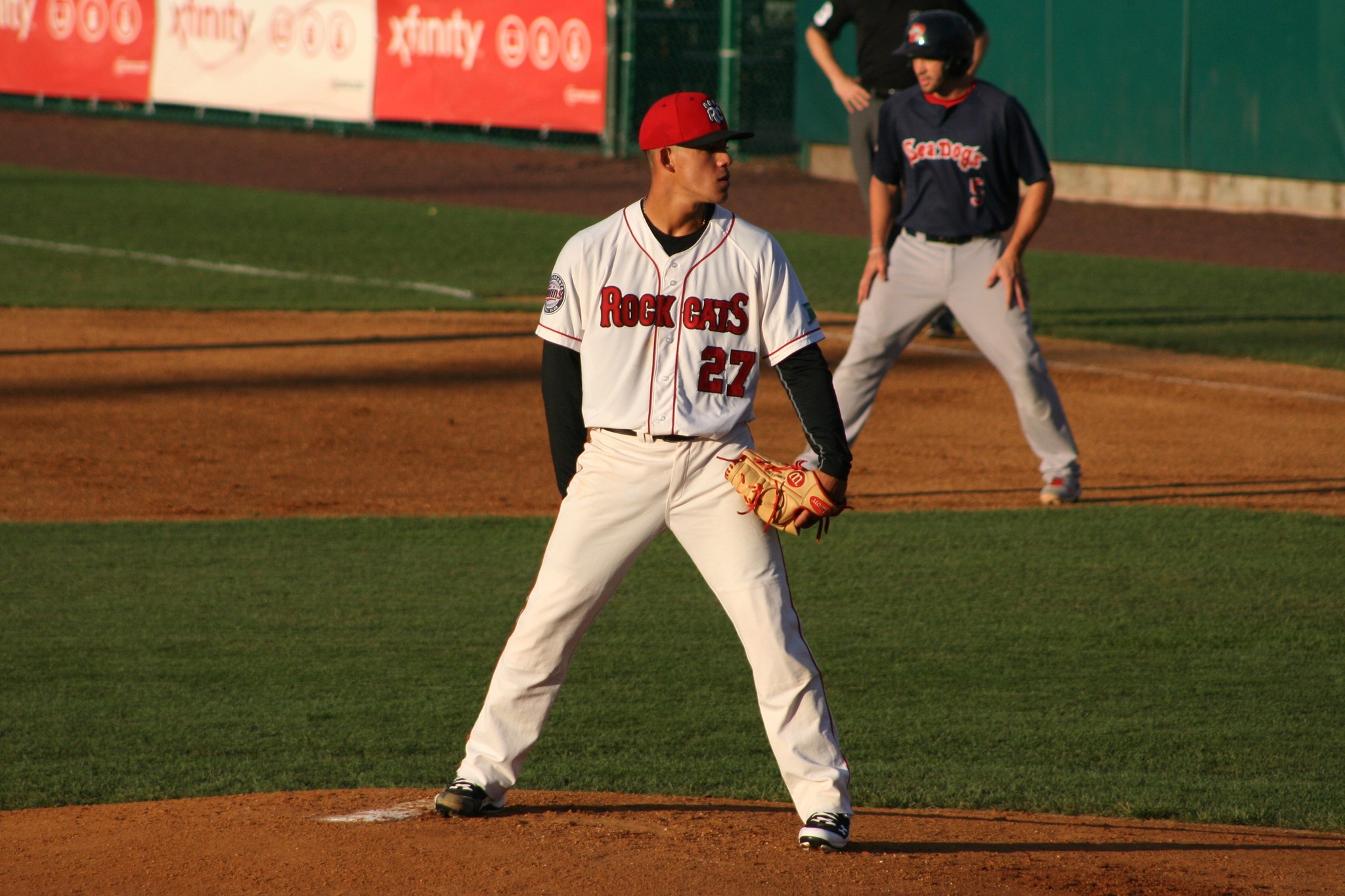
796;813;850;853
1038;480;1080;505
435;783;506;814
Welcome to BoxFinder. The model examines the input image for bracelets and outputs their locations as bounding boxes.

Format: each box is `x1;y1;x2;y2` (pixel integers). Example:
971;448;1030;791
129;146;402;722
868;248;887;255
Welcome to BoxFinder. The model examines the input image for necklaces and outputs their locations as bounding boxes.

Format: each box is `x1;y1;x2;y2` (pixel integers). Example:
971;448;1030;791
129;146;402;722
642;195;708;228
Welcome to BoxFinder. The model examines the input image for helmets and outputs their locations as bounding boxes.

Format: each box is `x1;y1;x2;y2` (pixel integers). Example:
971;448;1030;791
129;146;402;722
890;10;976;78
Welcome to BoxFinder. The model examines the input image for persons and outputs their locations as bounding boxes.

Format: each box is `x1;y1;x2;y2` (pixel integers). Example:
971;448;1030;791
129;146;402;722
794;11;1083;508
805;0;988;340
435;88;855;852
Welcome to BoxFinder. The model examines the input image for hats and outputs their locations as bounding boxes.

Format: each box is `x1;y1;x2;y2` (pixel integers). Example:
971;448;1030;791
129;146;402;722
639;91;755;148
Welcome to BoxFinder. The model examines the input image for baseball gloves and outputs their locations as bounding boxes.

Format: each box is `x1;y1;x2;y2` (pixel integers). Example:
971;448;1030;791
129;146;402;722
724;448;847;536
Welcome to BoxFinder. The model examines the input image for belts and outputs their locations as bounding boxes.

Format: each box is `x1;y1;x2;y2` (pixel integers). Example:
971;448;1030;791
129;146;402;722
604;428;693;442
906;225;969;244
